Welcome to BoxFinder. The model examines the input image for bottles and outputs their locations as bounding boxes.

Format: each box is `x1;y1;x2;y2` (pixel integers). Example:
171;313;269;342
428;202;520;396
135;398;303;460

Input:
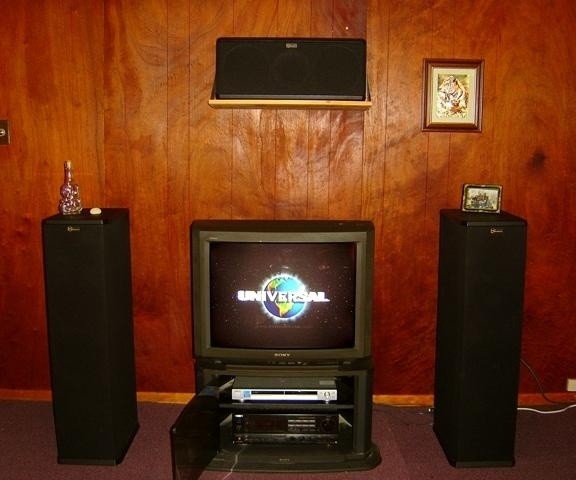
59;160;82;216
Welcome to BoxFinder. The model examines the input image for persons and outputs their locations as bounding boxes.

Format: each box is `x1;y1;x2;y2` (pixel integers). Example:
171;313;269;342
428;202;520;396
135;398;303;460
439;75;466;111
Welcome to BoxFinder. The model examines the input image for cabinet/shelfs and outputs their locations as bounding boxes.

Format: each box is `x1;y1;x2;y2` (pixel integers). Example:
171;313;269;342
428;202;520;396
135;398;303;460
169;363;383;480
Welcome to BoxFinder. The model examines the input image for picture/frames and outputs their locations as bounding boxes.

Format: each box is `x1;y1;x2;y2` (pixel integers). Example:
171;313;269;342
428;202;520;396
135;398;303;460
461;183;503;214
421;57;485;133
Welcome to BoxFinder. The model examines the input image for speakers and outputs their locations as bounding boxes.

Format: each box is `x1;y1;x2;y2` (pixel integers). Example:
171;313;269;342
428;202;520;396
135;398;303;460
215;36;367;100
432;210;526;469
42;209;135;465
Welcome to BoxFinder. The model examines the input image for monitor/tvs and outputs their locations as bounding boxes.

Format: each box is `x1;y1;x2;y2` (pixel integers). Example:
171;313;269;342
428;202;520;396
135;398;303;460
188;217;375;367
